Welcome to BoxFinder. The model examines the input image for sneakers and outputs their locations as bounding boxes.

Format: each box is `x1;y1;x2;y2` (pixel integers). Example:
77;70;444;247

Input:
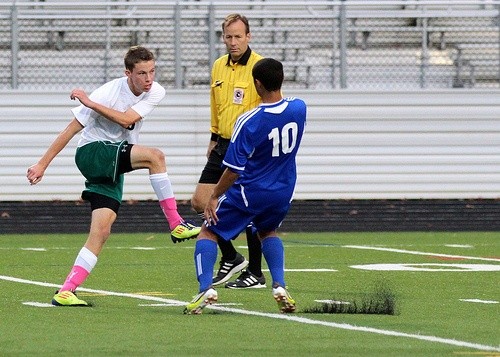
171;221;202;243
51;290;88;307
273;282;297;313
183;287;218;314
210;251;266;288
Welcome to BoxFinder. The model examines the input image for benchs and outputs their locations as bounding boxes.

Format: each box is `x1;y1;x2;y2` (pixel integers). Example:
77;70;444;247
0;0;500;91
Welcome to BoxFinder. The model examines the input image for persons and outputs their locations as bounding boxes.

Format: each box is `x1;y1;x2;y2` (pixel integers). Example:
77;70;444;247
191;12;283;289
183;58;307;315
26;47;202;306
